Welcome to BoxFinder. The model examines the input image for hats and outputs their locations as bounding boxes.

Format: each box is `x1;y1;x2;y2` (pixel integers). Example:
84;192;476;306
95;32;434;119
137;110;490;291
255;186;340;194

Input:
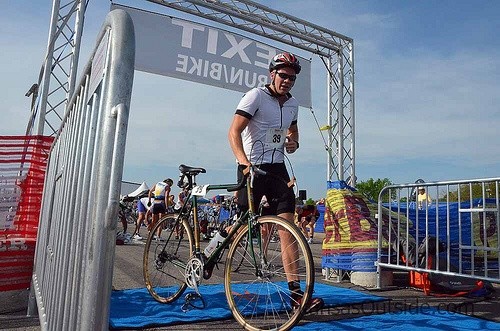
418;187;425;191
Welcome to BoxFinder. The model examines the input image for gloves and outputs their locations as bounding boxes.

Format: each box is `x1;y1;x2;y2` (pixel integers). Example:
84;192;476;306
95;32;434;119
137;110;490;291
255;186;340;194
165;209;168;212
147;201;151;207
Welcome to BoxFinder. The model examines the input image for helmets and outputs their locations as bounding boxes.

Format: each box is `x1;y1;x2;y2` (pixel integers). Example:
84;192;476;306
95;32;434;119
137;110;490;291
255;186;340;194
269;52;301;74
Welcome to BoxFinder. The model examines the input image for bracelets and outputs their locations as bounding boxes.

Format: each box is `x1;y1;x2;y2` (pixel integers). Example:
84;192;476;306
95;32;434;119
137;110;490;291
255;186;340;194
294;141;299;149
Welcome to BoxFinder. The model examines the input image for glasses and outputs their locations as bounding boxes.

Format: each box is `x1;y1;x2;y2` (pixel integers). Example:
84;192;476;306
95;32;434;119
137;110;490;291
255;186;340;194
277;72;296;82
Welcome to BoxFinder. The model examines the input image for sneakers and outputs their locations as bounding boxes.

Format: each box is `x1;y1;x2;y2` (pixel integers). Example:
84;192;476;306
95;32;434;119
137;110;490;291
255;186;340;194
203;261;216;280
292;297;324;316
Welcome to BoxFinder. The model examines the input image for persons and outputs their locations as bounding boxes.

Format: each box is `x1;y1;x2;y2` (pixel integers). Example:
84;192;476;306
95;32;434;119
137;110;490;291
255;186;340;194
418;186;431;210
202;52;324;317
120;178;328;244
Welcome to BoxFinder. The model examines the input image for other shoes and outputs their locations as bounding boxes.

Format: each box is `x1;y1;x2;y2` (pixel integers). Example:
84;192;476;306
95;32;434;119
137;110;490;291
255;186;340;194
133;233;143;239
306;238;313;244
153;235;164;241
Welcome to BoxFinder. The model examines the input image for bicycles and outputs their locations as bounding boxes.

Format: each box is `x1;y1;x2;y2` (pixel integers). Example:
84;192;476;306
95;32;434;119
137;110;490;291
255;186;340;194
142;139;315;331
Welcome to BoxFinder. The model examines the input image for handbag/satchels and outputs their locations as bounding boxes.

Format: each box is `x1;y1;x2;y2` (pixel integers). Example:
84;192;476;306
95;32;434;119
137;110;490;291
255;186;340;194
422;254;494;297
